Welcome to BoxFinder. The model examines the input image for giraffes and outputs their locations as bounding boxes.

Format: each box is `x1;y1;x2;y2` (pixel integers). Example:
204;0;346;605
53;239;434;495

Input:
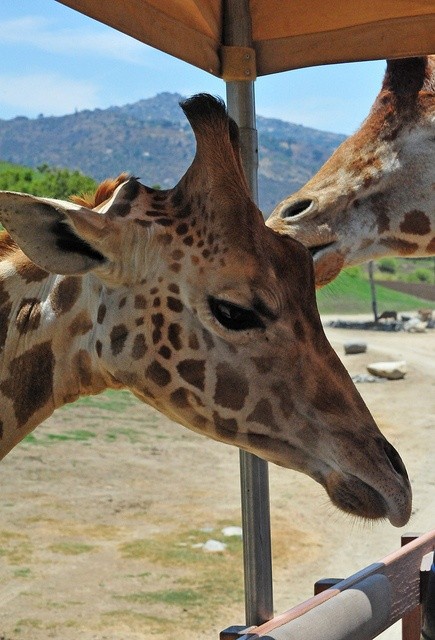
0;92;412;526
264;54;435;291
